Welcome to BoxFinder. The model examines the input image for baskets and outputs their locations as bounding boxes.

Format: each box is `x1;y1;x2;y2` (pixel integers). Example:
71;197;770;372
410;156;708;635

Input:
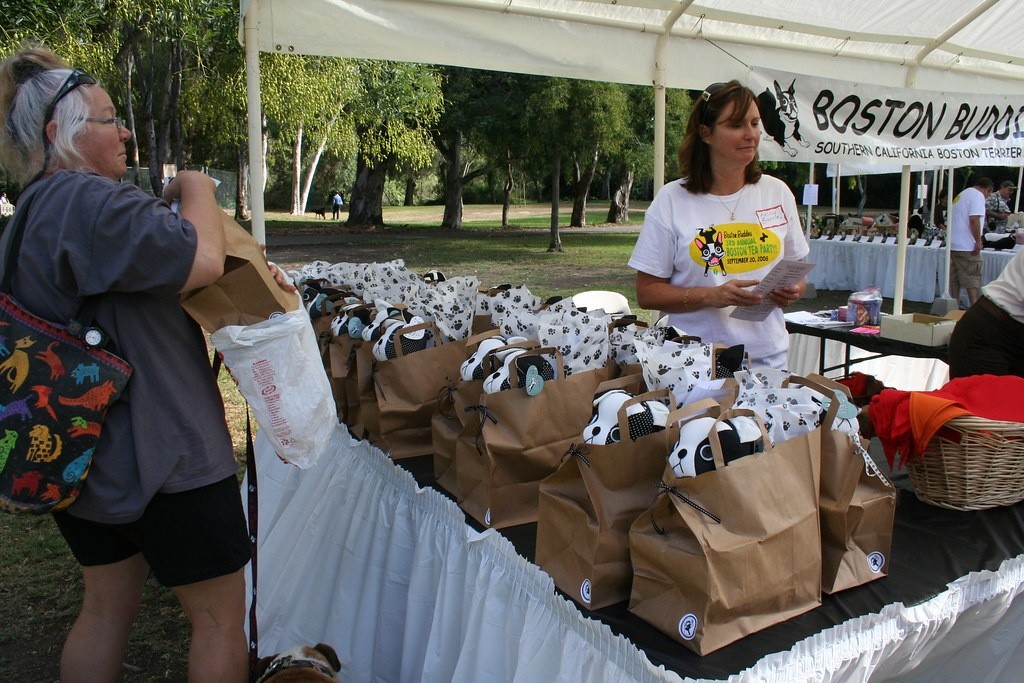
907;414;1024;511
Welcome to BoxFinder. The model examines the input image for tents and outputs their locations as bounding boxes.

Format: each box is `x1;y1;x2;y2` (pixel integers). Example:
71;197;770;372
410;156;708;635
237;0;1024;318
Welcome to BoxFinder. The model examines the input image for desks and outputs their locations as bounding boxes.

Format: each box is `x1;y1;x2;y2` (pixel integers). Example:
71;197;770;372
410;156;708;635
804;235;1024;314
235;417;1024;683
784;308;959;394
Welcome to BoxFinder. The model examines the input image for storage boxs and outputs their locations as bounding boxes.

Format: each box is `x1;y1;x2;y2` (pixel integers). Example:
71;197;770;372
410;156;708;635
875;309;964;347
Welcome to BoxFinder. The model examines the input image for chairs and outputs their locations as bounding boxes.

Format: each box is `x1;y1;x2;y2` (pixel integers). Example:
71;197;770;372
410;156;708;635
572;290;631;317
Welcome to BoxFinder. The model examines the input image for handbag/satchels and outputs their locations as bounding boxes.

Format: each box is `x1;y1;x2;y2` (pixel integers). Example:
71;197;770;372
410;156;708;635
179;206;339;468
286;260;896;655
0;193;133;515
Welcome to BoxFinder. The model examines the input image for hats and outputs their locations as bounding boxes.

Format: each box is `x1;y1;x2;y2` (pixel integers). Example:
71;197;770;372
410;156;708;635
1000;181;1018;189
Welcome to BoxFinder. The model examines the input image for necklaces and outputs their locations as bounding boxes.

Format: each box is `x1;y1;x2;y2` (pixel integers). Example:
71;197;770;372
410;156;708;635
712;183;745;220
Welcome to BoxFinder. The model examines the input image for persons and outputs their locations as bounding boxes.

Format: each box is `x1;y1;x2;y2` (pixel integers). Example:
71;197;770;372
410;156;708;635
627;79;811;370
331;190;342;220
0;192;9;204
0;47;297;683
949;177;994;311
934;181;1018;233
948;244;1024;381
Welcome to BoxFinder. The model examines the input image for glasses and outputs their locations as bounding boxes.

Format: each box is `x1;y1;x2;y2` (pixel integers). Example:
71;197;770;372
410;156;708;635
701;83;726;119
44;68;96;121
78;117;125;129
